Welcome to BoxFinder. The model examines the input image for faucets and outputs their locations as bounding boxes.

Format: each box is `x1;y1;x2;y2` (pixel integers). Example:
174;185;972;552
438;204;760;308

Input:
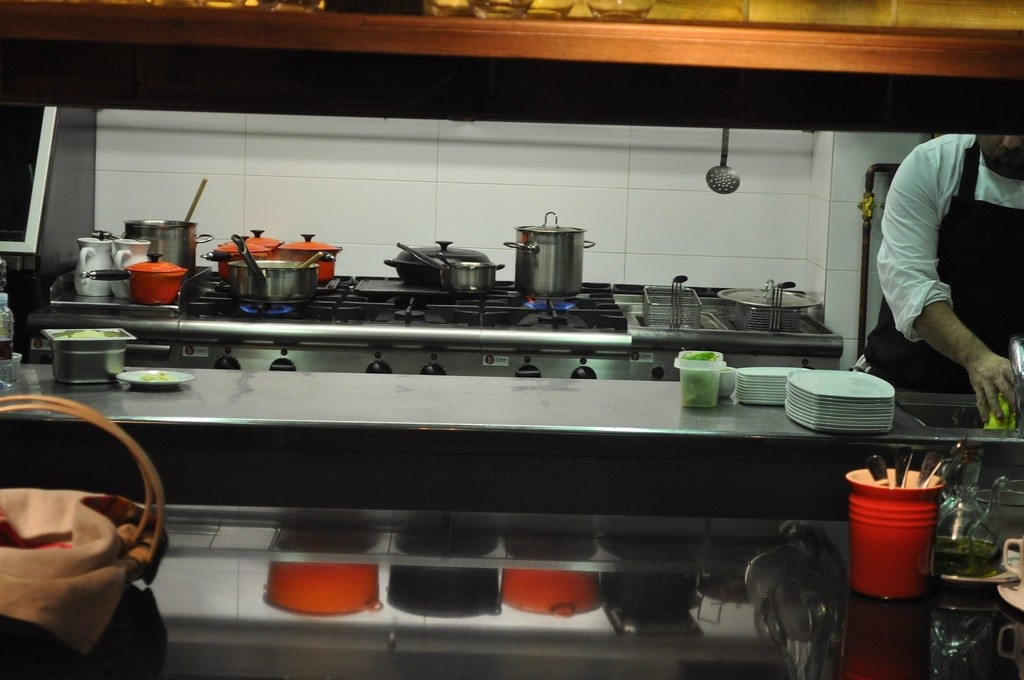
1008;336;1024;437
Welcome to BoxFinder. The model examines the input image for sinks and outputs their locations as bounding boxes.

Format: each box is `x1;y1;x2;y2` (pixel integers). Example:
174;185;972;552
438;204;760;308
897;402;1024;430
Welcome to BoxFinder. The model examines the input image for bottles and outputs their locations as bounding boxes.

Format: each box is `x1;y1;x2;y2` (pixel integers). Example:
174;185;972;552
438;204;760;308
0;292;14;387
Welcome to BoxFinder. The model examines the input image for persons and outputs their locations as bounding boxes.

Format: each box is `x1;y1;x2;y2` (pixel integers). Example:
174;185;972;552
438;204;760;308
859;134;1024;424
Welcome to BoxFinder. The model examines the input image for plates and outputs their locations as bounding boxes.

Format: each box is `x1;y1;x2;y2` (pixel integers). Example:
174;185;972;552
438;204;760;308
940;574;1019;581
735;355;896;433
115;370;195;389
996;584;1024;612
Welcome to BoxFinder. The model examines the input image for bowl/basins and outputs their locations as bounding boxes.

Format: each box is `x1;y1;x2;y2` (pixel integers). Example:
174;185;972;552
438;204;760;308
671;350;726;407
123;253;187;304
718;366;736;397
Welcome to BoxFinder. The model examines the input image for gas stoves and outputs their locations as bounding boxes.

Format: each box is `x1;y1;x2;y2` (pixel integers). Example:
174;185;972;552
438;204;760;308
188;279;633;355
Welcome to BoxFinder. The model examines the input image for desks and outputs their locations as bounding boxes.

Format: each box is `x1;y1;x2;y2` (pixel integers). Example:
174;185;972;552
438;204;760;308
132;503;1024;680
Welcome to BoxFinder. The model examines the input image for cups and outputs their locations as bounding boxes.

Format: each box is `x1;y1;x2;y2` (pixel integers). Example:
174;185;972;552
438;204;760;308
11;353;22;380
1002;536;1024;586
113;239;149;298
845;468;945;598
74;237;112;296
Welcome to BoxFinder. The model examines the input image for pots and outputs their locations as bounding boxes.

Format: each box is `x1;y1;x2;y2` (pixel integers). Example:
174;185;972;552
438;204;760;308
384;240;505;293
503;211;596;299
121;220;215;287
201;230;343;303
